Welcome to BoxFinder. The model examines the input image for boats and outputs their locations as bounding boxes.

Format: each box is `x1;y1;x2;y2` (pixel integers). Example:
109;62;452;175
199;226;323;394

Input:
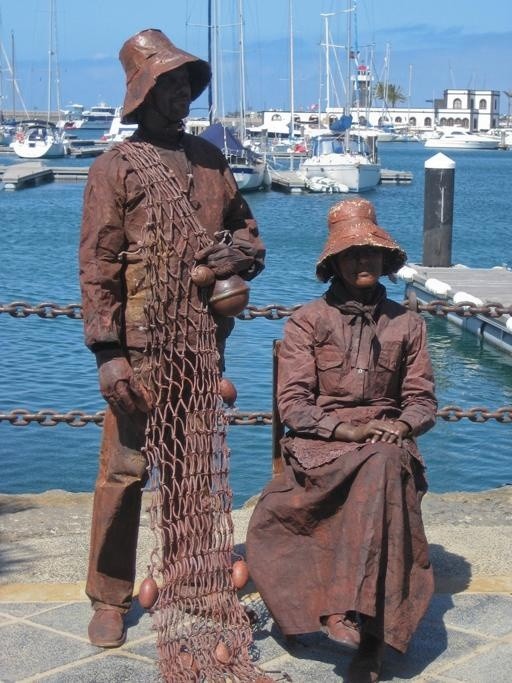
0;0;139;159
247;0;512;151
305;176;349;194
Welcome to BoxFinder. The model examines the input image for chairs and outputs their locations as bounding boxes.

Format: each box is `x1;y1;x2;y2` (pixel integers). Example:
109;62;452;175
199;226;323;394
272;339;428;645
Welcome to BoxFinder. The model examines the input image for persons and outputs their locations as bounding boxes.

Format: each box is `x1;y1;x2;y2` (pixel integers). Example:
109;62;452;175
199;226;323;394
80;27;265;645
255;196;434;683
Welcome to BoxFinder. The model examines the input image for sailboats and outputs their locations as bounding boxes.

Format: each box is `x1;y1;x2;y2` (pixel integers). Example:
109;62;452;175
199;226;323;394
297;0;382;193
184;1;268;193
